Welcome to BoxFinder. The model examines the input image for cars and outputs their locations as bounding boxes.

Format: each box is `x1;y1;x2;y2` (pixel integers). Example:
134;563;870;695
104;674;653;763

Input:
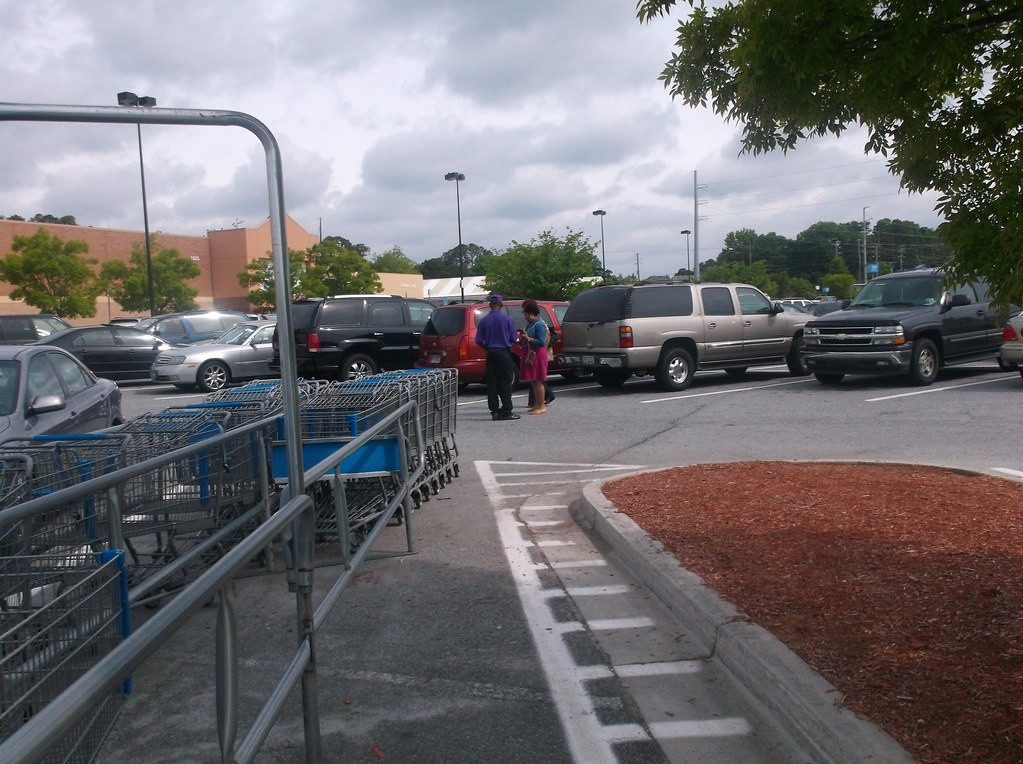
246;313;277;321
24;324;187;380
737;293;848;317
149;320;278;394
1000;311;1023;382
0;345;127;461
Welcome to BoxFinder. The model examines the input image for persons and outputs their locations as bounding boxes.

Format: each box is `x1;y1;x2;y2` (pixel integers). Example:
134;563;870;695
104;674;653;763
475;294;521;420
518;299;555;415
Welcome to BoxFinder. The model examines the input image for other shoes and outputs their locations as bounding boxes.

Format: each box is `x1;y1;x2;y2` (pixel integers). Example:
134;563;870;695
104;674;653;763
528;402;535;406
530;408;547;415
528;408;534;412
500;413;521;420
492;413;500;419
544;396;555;405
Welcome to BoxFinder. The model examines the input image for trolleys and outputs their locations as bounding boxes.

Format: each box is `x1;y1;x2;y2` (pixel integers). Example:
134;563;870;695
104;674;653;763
0;368;462;764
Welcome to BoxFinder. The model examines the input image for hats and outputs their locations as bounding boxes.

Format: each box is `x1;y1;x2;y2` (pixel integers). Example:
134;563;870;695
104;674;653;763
490;294;502;303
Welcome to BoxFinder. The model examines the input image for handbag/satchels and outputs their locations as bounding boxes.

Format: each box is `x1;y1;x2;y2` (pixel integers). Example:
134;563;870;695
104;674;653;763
524;341;536;365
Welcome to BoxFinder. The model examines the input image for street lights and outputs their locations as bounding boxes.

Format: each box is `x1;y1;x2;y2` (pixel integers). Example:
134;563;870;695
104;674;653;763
444;171;466;303
681;230;691;282
117;92;158;318
593;209;607;286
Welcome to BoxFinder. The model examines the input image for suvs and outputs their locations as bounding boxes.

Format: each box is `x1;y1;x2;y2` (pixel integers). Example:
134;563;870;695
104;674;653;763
800;267;1023;387
131;309;251;346
413;299;596;394
556;280;819;393
268;294;436;382
0;313;72;346
109;316;143;329
